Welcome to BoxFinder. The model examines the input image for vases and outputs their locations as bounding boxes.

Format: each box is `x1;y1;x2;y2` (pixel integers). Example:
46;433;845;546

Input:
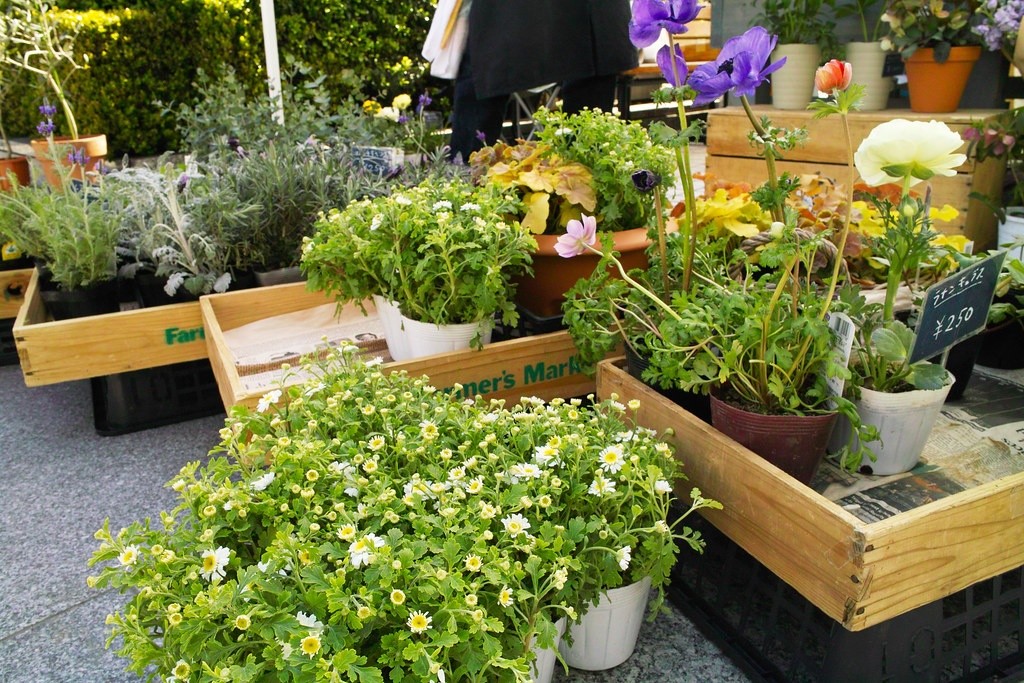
558;575;650;670
901;47;981;114
399;312;494;360
529;617;564;683
828;345;955;477
136;273;232;307
975;288;1024;370
371;293;412;361
894;311;981;402
512;228;653;317
705;374;840;486
255;267;307;286
36;274;120;321
622;334;712;424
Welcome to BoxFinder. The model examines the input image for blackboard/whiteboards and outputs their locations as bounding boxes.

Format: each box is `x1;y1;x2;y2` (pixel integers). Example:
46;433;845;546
350;142;405;181
905;248;1009;370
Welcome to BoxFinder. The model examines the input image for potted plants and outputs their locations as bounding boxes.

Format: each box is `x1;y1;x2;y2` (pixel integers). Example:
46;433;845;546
0;10;30;191
821;0;893;111
0;0;108;188
747;0;837;111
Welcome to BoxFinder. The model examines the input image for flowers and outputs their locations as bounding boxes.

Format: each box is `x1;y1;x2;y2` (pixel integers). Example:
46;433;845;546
852;198;1024;333
371;172;540;352
0;95;130;292
107;161;232;297
466;100;678;234
641;25;884;475
878;0;1024;63
552;0;751;377
183;133;370;272
83;333;725;683
839;117;967;391
299;194;388;324
961;106;1024;260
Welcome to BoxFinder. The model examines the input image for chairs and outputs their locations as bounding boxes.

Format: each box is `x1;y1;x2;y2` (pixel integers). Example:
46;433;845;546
616;0;725;147
